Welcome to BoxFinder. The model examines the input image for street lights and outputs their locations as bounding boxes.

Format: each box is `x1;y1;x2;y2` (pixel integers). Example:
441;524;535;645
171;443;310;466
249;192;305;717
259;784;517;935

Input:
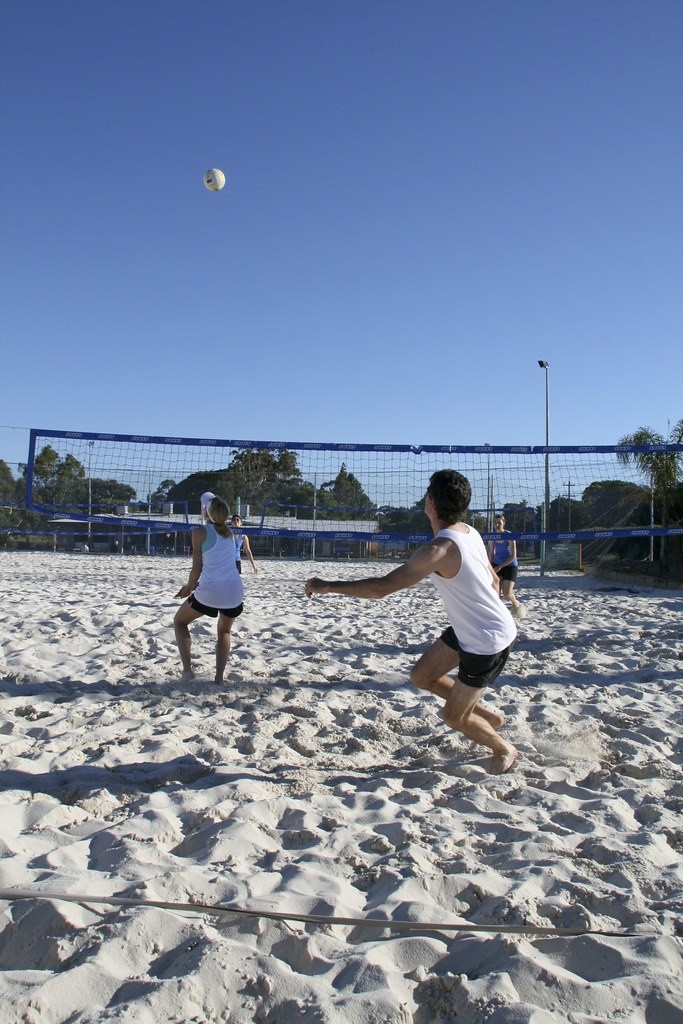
482;443;491;542
88;440;95;545
537;359;549;577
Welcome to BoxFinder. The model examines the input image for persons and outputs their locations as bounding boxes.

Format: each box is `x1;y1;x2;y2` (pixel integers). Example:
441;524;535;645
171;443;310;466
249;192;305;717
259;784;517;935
305;469;517;775
231;515;257;574
174;492;244;685
80;542;89;553
489;514;526;619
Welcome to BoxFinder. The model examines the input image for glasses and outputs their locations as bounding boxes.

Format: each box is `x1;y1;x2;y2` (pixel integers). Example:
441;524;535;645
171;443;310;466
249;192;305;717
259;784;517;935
232;518;240;521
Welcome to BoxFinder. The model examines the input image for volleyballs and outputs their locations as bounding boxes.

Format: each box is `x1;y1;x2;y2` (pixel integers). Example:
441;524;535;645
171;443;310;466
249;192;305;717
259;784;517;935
203;168;226;192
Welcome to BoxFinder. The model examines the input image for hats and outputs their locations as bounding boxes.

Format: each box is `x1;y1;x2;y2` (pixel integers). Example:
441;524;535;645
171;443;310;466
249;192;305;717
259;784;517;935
201;492;217;523
